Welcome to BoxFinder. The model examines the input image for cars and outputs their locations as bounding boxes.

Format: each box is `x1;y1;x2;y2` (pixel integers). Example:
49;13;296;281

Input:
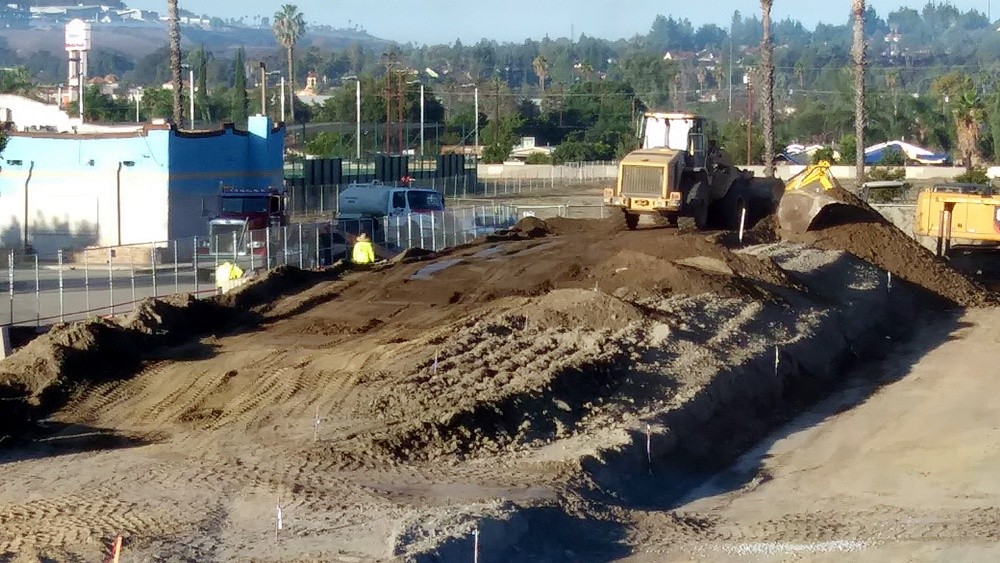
469;213;519;239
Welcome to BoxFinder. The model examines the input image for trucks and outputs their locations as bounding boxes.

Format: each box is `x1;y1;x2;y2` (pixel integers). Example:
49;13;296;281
192;184;331;282
334;177;446;244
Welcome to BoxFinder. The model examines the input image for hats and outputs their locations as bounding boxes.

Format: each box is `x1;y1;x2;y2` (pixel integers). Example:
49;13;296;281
356;233;370;242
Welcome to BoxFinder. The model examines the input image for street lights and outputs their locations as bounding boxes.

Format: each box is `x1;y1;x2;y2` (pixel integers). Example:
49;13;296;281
181;63;195;130
345;75;361;163
412;79;425;157
461;82;479;153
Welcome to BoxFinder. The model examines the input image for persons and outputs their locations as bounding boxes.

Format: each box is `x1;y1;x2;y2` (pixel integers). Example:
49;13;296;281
353;234;374;264
215;262;232;296
267;185;279;214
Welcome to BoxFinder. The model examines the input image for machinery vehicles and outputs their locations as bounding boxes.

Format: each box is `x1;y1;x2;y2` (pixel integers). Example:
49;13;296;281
783;158;860;203
603;110;785;231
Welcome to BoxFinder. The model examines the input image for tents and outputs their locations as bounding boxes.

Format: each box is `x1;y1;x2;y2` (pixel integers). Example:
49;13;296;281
862;140;947;166
